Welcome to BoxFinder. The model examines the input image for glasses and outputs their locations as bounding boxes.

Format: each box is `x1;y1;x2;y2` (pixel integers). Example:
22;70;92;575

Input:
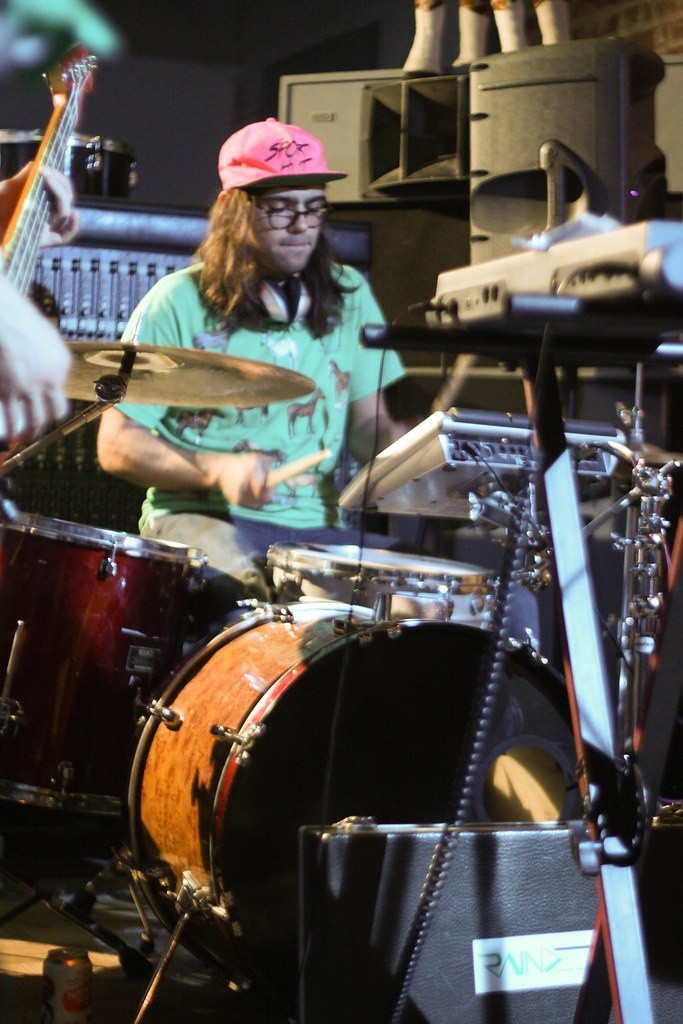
249;206;334;230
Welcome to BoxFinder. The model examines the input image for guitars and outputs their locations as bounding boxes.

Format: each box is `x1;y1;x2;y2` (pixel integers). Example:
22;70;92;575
0;43;100;469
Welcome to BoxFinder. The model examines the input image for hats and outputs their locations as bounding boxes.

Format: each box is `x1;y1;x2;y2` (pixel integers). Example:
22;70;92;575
217;117;348;191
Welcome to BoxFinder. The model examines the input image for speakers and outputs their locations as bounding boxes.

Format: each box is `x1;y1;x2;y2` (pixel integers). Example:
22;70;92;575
359;74;471;197
468;37;668;270
295;821;599;1024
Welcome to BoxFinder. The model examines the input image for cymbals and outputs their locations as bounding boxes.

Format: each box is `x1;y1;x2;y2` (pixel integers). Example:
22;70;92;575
61;339;316;408
586;439;683;464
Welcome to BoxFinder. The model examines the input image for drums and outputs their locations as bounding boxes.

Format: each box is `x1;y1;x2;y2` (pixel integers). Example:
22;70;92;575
1;128;138;202
2;507;212;819
125;597;588;1015
265;541;499;634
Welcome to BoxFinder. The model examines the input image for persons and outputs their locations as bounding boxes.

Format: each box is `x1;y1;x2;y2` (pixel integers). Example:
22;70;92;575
96;118;540;659
0;161;79;444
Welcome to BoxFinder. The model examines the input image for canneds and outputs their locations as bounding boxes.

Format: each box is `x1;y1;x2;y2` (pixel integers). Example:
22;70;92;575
42;947;94;1024
